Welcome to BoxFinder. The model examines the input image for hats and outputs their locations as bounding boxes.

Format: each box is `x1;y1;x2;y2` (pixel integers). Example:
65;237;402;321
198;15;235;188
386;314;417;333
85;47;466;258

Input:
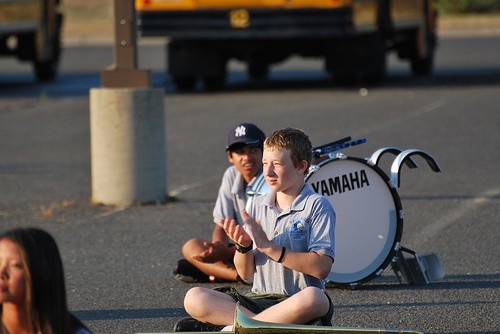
225;123;266;152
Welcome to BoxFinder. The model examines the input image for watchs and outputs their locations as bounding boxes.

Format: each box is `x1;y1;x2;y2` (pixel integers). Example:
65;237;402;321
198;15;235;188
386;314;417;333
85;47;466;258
235;239;253;254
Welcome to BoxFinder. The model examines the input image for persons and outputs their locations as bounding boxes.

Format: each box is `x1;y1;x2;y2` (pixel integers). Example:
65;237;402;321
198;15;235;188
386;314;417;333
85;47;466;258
171;123;272;286
0;226;93;334
174;129;336;333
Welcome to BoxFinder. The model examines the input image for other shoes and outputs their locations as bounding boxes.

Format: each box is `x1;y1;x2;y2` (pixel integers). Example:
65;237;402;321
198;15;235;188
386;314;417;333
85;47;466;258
173;259;209;284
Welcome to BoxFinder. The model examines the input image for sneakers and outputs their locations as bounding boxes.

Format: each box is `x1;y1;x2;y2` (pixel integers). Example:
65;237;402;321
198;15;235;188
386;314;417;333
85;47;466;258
174;317;225;332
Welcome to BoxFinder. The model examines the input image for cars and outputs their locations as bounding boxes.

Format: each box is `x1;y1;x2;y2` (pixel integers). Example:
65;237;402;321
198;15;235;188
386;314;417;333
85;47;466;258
0;1;65;86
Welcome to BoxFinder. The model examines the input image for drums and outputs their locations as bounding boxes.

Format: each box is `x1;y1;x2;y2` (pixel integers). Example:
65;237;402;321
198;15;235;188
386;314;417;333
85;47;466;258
301;152;405;287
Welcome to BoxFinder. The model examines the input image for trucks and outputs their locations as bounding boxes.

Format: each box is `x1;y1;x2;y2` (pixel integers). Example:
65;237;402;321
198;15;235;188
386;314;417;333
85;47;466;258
135;0;440;89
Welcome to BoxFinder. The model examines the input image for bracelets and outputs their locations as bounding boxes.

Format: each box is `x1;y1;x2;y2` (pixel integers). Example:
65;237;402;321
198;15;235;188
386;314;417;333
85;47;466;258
276;246;286;263
